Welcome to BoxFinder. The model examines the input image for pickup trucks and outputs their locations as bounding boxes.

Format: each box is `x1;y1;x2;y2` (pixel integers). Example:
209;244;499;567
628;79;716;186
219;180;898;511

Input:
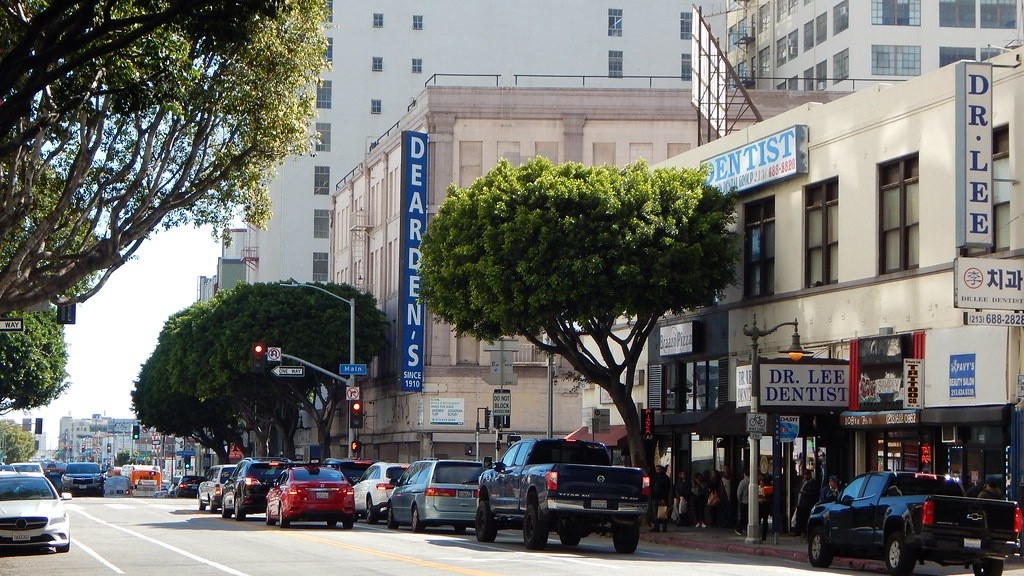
475;438;651;554
808;470;1022;576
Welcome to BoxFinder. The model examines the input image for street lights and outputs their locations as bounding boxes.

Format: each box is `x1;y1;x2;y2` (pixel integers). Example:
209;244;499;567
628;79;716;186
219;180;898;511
280;283;354;458
744;314;814;543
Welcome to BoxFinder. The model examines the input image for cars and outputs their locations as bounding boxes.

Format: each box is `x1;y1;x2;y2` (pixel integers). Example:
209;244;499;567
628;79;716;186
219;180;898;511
0;461;107;552
154;475;204;499
264;466;355;529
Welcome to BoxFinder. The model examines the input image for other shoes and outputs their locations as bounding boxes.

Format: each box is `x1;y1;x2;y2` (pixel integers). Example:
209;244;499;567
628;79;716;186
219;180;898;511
790;533;800;536
702;524;706;528
662;527;667;532
695;523;700;527
800;534;806;538
650;528;660;533
735;530;742;535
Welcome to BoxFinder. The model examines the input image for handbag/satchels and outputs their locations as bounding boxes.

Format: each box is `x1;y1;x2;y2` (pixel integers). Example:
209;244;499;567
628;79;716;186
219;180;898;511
791;508;798;528
671;498;680;523
657;502;667;519
679;497;687;513
708;491;718;506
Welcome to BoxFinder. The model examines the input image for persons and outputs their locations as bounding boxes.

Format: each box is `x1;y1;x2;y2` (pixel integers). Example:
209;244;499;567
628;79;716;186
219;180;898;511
792;469;840;538
977;478;1006;500
674;465;731;528
756;470;769;543
735;471;749;535
648;465;672;533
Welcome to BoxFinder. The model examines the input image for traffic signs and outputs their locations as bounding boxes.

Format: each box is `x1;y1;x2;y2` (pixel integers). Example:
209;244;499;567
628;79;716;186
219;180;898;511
271;366;305;377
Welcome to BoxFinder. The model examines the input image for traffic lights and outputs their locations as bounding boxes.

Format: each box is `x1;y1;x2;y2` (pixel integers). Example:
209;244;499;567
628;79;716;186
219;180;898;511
178;460;181;468
68;445;72;454
133;425;139;440
350;400;364;429
184;457;191;470
82;445;85;453
252;342;264;372
507;435;521;447
486;410;490;428
351;440;361;451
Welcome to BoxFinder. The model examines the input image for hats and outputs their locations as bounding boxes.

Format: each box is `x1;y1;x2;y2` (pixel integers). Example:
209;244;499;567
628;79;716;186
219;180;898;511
829;475;838;482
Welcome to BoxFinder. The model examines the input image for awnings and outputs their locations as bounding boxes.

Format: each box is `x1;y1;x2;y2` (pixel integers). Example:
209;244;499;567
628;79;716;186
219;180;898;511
697;403;830;474
562;424;633;446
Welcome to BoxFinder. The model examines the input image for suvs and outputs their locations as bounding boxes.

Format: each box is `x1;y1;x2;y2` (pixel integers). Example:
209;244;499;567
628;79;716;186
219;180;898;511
323;457;411;525
389;457;482;535
198;464;242;513
222;458;292;521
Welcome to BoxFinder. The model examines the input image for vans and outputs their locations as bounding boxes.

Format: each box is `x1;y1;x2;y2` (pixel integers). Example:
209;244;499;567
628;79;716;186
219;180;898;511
104;477;130;498
